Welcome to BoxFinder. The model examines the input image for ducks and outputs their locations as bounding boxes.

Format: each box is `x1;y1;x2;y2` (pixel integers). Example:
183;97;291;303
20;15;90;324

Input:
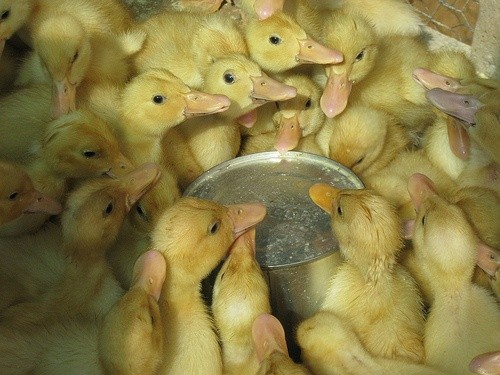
0;1;500;375
